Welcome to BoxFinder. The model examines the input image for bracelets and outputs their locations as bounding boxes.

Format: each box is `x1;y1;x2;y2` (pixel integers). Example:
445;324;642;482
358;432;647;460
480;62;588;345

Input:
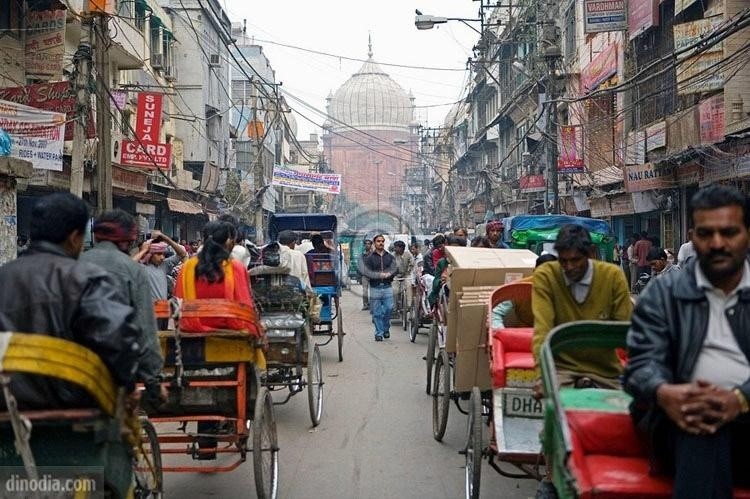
731;384;748;415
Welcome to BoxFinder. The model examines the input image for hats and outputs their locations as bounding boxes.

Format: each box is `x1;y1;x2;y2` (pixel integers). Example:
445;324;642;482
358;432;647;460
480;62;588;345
278;230;298;245
486;220;504;233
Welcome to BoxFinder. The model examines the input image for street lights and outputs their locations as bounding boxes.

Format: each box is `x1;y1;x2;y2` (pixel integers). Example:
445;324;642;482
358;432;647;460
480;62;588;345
383;118;456;229
411;14;563;216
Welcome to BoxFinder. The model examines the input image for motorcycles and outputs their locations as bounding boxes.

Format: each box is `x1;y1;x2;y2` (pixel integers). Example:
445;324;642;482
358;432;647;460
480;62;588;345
334;232;372;284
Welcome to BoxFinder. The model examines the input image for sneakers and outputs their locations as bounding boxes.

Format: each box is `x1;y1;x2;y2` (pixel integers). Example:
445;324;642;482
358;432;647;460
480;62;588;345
375;336;383;341
383;330;390;338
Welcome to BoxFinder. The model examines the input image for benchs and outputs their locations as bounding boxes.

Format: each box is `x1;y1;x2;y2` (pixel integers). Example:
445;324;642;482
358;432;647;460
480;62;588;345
559;409;750;496
488;328;632;387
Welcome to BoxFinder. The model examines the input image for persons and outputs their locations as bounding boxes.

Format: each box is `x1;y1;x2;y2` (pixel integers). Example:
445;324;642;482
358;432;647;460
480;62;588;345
621;180;748;498
